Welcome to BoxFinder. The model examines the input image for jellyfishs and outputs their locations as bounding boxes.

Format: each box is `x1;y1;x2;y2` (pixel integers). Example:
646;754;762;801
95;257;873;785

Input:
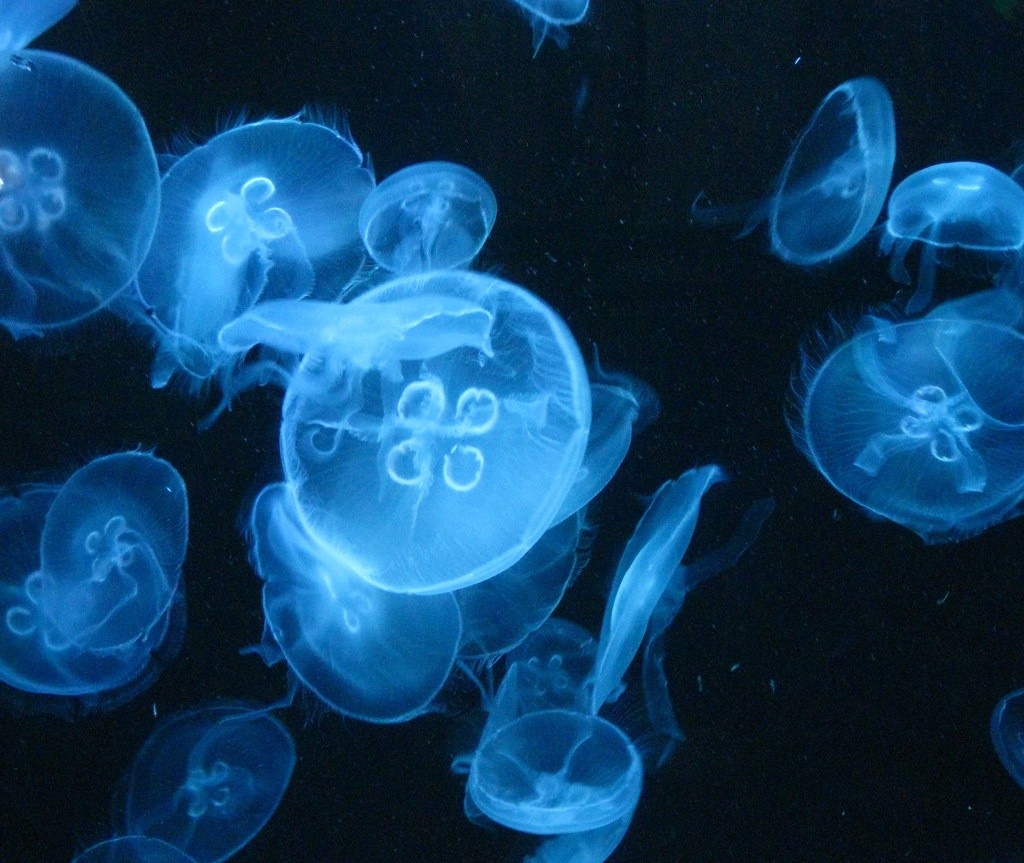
0;0;1024;863
